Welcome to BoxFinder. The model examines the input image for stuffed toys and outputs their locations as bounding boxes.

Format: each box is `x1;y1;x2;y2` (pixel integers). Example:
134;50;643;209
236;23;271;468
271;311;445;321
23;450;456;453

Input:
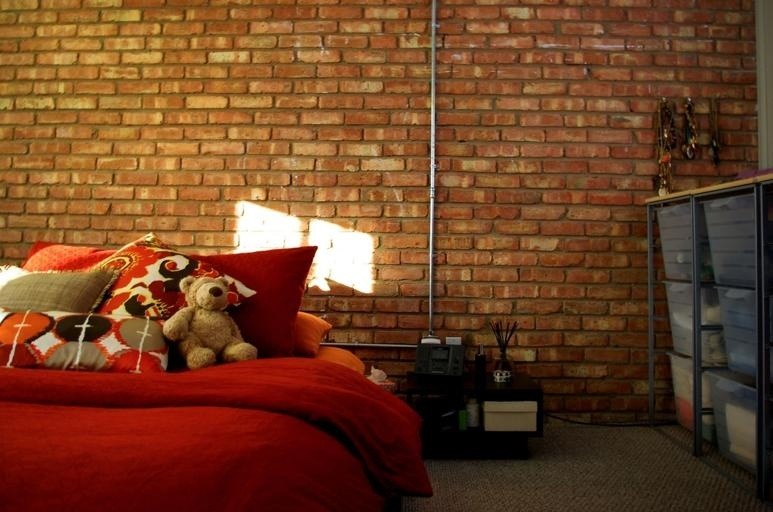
163;275;257;369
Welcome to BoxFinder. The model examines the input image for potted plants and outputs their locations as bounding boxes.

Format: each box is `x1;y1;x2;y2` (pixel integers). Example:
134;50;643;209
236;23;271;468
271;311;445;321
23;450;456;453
490;317;518;385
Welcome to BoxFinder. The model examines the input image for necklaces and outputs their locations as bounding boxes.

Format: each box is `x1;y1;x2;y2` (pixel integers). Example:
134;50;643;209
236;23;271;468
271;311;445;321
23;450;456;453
656;92;722;197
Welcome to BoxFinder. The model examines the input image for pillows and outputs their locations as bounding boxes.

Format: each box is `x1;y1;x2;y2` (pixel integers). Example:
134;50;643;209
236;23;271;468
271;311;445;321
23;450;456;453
85;233;256;323
0;265;121;315
19;240;320;362
1;310;173;374
291;309;334;357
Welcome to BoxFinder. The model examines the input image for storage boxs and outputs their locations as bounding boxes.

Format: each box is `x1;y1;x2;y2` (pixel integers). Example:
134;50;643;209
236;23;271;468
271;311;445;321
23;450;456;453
654;202;716;282
712;373;755;475
661;277;727;366
666;351;715;446
701;192;758;289
713;285;756;376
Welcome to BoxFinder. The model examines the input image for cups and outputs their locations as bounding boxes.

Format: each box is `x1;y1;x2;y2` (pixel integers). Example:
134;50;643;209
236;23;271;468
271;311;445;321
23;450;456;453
474;353;485;370
459;409;466;430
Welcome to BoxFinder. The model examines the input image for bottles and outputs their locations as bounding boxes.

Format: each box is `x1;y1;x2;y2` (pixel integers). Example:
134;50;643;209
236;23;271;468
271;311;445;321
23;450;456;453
466;398;479;428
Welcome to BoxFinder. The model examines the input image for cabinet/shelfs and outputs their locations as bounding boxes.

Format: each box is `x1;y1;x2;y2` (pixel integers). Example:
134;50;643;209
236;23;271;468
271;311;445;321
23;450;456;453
480;369;545;462
643;169;770;502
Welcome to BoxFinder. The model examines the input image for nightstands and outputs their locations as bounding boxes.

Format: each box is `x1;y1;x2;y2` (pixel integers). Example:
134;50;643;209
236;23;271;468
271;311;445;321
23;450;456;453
404;355;479;460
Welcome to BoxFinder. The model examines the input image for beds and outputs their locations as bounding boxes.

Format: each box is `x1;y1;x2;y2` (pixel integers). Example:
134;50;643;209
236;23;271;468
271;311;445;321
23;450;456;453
0;233;434;512
